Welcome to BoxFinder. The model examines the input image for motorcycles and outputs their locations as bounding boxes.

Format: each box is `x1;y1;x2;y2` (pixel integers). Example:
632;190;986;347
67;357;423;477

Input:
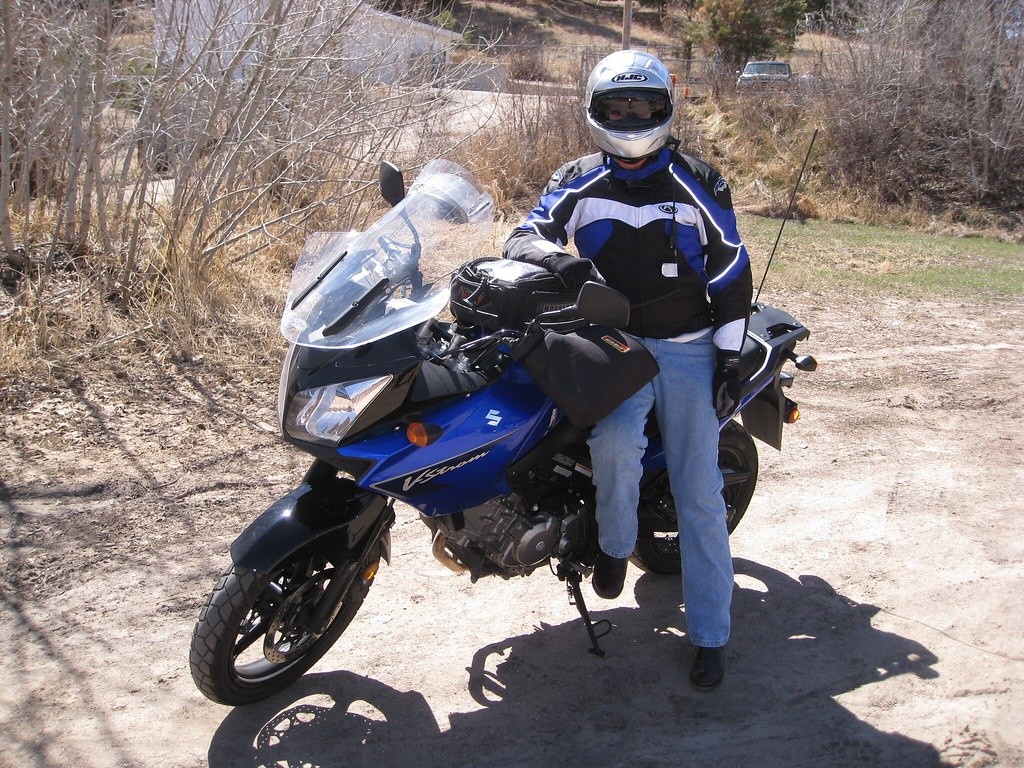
186;158;819;707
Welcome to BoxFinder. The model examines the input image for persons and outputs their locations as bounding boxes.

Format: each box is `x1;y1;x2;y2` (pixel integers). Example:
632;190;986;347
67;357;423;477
501;50;753;691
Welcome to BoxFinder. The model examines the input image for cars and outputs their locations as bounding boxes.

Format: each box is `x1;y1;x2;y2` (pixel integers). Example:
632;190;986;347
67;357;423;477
734;61;799;98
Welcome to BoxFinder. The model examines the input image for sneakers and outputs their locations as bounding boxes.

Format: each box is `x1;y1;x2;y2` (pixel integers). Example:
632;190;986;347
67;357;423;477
592;550;628;599
689;645;725;690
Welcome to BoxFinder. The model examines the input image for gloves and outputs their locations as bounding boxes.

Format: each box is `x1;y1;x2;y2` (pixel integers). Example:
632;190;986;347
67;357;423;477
713;348;742;420
548;252;606;292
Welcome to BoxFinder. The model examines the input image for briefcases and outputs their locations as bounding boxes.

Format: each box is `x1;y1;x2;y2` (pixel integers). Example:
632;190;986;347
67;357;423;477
450;257;586;332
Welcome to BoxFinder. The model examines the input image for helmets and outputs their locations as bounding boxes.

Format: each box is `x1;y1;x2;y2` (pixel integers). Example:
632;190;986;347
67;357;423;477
585;49;675;159
